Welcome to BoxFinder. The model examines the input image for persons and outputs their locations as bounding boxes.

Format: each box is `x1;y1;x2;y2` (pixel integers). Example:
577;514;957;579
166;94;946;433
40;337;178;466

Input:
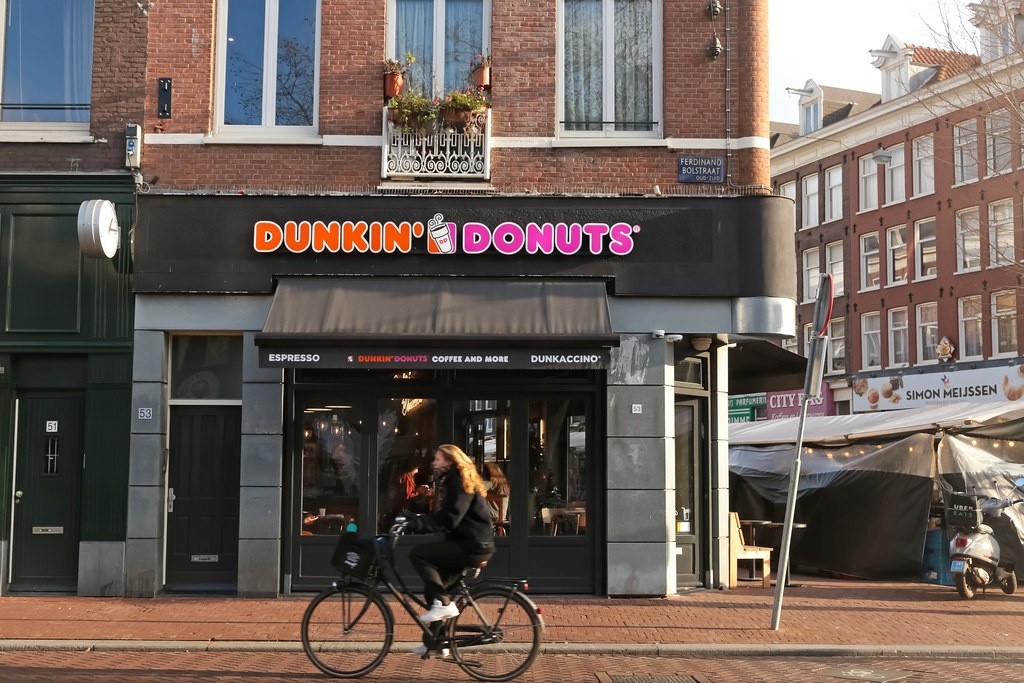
396;444;494;659
399;460;430;530
482;460;510;529
545;472;556;498
547;492;567;536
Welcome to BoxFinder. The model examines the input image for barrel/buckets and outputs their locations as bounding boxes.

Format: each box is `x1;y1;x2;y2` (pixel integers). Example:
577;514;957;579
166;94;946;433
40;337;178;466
922;530;958;586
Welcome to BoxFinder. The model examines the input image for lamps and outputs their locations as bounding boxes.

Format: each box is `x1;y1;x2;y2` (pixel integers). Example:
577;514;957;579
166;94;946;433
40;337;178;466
898;371;905;376
871;146;892;165
949;366;956;371
1008;359;1016;368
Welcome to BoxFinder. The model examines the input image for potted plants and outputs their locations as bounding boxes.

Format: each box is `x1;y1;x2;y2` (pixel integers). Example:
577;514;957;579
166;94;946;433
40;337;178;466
387;93;439;135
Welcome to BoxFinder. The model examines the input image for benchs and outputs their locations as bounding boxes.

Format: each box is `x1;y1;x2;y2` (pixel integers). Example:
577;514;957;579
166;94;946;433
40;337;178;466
730;511;775;589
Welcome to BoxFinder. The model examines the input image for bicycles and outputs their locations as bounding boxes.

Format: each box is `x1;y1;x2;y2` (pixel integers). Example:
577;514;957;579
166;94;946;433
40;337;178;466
301;513;545;683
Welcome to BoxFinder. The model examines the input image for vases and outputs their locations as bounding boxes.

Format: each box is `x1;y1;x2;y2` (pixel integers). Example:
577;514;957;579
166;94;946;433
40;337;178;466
441;106;488;123
384;73;405;97
473;66;491;92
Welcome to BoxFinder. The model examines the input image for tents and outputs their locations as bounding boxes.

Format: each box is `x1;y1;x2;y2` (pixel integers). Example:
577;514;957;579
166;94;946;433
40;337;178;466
484;432;585;455
728;399;1024;583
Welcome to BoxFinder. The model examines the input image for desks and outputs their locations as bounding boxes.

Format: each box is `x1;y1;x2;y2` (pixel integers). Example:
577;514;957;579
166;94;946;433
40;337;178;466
766;523;807;588
738;519;772;581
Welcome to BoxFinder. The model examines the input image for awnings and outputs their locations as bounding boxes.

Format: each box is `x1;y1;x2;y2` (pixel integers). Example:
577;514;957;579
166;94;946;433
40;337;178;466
254;276;620;345
725;337;809;392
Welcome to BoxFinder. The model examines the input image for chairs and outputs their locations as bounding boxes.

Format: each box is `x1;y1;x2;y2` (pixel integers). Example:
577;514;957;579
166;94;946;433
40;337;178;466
549;514;581;536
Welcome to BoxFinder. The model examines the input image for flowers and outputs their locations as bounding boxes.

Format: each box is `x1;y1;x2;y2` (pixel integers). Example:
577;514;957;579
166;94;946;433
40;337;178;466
435;85;489;110
469;53;486;71
383;52;415;74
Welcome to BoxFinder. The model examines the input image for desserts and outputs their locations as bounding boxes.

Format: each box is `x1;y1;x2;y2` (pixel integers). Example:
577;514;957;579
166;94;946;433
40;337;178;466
867;390;879;409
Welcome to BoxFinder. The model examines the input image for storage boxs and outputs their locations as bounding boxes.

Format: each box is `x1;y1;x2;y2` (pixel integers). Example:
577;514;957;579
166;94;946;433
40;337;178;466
924;530;955;586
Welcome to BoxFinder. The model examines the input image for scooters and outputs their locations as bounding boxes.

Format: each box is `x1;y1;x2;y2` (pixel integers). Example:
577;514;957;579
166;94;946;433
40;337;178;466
947;476;1024;601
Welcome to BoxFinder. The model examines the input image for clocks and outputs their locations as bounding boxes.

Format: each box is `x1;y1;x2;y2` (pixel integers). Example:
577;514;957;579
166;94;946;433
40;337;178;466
98;200;119;258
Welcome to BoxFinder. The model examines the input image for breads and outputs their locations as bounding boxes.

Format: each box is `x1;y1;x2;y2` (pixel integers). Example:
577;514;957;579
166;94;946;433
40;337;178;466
1002;376;1024;401
881;383;893;398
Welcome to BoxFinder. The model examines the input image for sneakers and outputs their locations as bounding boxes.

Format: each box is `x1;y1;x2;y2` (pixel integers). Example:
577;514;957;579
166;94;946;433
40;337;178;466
412;643;450;661
417;597;460;623
